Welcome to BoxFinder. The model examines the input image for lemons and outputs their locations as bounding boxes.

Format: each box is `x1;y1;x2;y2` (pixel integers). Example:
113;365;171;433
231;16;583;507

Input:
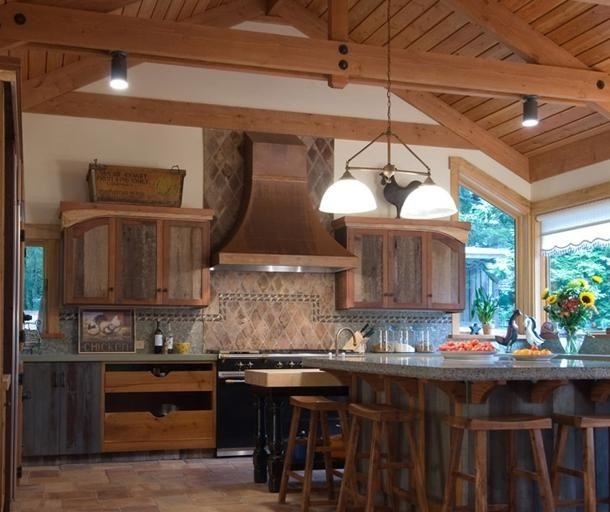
512;345;551;357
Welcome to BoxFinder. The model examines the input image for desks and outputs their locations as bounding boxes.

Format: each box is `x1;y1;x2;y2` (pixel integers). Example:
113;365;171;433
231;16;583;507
244;370;351;494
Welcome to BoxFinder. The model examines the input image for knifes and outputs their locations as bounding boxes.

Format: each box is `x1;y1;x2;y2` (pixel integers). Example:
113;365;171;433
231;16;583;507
360;322;376;338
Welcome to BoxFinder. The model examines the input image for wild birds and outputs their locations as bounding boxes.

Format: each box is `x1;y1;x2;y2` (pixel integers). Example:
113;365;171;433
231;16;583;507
495;309;523;353
523;316;546;350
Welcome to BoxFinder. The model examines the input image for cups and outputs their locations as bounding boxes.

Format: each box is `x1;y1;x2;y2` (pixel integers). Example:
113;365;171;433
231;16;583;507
371;328;436;354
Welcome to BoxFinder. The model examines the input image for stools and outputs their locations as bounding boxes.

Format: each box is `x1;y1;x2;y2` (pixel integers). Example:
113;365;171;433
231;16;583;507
439;413;551;508
554;413;609;511
333;401;432;508
280;396;349;512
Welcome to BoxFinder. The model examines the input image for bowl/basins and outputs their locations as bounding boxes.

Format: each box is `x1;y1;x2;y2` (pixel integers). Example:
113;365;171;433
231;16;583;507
173;342;191;354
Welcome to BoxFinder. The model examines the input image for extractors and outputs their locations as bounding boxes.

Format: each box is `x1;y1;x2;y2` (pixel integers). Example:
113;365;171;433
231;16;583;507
211;130;360;274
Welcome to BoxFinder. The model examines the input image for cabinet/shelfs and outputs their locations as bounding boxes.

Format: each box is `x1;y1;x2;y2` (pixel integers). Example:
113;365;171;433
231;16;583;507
19;361;101;459
386;219;471;313
334;218;386;310
101;358;217;458
114;204;222;305
56;203;114;307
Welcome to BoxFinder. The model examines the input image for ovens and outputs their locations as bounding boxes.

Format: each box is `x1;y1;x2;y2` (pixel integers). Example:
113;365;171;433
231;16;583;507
214;377;356;458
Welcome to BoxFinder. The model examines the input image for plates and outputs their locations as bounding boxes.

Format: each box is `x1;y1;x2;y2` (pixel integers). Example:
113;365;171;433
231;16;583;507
439;351;497;358
511;362;556;371
440;358;497;368
509;352;557;362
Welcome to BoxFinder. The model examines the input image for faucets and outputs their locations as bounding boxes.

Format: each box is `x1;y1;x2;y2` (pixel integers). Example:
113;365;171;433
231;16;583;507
335;327;358;357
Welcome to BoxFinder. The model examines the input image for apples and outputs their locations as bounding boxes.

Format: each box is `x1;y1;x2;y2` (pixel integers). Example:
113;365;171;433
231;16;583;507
439;339;494;352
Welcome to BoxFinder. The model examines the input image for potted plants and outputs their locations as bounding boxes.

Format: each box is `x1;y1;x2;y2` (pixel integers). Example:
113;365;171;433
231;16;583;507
470;287;499;334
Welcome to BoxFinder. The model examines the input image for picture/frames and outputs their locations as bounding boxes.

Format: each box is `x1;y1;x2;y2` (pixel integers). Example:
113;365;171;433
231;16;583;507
78;307;137;354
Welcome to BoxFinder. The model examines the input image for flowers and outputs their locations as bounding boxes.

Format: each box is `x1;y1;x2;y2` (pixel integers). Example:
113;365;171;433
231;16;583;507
540;271;605;327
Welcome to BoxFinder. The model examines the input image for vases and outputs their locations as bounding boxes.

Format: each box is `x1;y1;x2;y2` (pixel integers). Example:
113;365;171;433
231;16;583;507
558;324;585;353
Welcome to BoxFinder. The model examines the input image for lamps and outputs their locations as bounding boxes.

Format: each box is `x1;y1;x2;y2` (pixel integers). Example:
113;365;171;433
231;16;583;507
521;95;539;127
316;0;464;220
108;48;129;90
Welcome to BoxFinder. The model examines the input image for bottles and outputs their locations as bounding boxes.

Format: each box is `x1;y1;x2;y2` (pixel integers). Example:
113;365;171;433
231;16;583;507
153;319;164;353
163;322;173;354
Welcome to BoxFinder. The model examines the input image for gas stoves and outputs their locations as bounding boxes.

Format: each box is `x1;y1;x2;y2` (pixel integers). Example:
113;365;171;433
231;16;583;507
208;347;361;377
